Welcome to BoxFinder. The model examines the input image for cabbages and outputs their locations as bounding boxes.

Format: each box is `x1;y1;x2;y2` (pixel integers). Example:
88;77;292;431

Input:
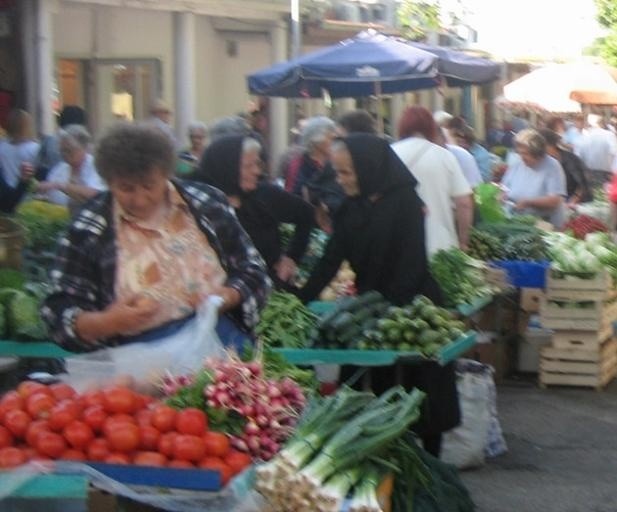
541;230;617;280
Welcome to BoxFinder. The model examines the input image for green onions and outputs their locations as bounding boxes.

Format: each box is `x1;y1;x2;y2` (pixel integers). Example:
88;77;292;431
255;384;436;512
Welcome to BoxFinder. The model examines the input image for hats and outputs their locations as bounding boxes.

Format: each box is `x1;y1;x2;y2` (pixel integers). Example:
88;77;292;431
147;97;173;114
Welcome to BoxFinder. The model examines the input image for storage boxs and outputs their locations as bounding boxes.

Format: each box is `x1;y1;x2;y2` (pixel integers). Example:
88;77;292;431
475;254;616;392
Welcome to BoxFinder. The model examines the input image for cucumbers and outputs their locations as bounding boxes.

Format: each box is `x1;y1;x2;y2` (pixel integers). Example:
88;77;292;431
306;291;390;350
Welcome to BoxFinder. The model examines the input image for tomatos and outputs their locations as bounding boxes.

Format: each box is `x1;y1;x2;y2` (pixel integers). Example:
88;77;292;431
0;380;251;490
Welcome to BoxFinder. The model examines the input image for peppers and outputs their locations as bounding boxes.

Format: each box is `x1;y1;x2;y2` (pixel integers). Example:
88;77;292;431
358;293;466;356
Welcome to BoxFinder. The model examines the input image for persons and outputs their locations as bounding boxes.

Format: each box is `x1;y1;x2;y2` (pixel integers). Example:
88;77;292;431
289;137;462;462
148;99;176;147
0;109;44;215
38;104;89;180
38;116;272;390
18;125;109;209
